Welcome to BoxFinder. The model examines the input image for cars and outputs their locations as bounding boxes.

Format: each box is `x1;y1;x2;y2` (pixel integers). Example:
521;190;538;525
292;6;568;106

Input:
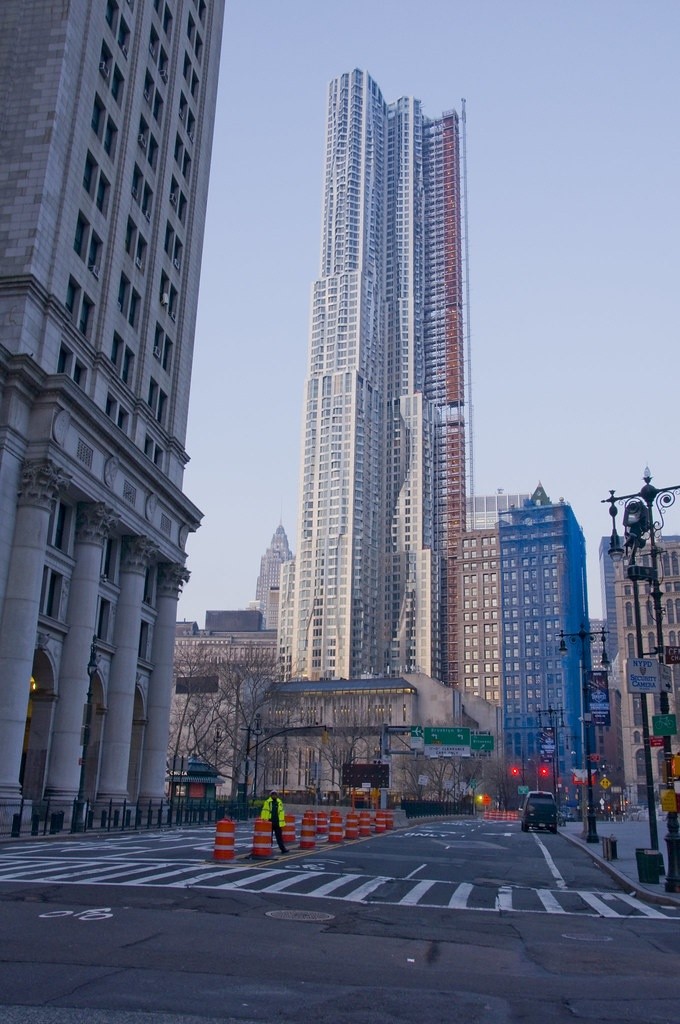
560;807;575;821
519;791;556;815
521;799;559;835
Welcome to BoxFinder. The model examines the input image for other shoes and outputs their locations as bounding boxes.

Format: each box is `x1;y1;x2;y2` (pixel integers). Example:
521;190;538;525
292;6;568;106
281;849;290;854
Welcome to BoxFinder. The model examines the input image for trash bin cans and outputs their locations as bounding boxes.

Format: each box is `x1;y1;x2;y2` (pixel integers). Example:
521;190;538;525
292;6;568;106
72;801;87;832
602;836;618;860
635;849;661;884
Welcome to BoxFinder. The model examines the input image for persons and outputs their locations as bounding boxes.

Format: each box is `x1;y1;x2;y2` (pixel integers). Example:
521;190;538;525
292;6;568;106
261;791;289;853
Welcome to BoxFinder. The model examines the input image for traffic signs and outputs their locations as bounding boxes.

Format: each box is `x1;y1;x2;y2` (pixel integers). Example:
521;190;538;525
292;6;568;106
423;726;471;757
660;664;673;694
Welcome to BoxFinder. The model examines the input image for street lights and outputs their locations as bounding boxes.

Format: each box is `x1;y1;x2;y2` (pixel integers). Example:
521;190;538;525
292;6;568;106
602;464;680;894
555;618;612;844
252;713;262;800
213;723;222;800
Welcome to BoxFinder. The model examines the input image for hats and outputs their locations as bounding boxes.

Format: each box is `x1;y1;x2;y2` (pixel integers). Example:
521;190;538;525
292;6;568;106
268;788;279;796
